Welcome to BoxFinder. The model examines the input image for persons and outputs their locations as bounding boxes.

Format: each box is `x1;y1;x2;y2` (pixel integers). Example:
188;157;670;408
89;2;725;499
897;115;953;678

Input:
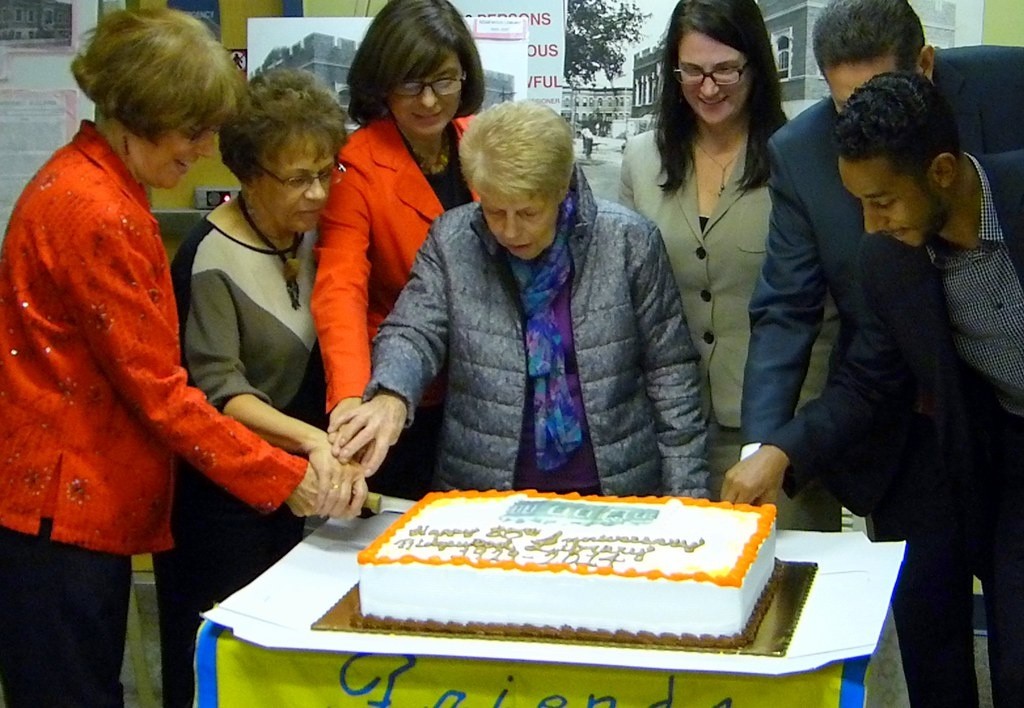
150;70;368;708
0;8;318;708
328;102;712;503
722;72;1024;708
581;125;594;158
309;0;485;497
618;0;846;532
740;1;1024;708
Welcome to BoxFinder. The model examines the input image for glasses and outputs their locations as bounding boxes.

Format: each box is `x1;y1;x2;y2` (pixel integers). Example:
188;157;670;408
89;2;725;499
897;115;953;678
252;158;348;193
392;69;467;97
672;62;748;86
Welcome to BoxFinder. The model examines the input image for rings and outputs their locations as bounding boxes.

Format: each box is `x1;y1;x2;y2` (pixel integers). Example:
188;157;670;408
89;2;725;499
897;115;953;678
330;484;340;488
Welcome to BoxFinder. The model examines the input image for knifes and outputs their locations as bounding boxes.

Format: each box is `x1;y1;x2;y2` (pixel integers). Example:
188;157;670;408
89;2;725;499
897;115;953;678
362;491;419;514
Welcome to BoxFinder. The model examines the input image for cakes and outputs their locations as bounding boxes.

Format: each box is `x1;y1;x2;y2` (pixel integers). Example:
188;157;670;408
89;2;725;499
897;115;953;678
357;488;778;648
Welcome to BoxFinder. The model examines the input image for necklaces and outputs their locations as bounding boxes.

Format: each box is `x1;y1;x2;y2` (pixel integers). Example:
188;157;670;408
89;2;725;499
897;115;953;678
695;138;739;195
238;191;302;310
